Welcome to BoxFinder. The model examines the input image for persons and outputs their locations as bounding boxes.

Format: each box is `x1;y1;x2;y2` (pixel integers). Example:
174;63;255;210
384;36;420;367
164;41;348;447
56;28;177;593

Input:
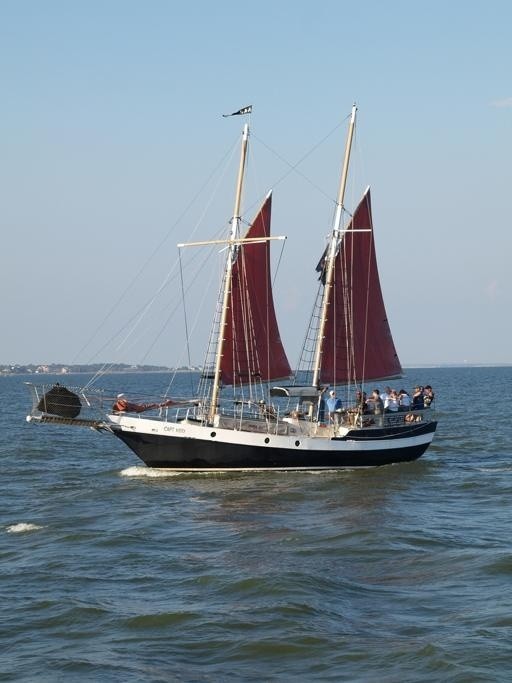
363;384;434;425
328;390;343;422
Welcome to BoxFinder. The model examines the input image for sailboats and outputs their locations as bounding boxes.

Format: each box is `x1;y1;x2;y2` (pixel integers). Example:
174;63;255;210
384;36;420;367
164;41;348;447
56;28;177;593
22;100;438;471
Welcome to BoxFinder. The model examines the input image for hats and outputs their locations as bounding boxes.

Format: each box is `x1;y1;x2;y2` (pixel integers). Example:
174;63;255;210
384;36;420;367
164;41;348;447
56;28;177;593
329;390;335;395
424;384;431;389
411;385;419;389
